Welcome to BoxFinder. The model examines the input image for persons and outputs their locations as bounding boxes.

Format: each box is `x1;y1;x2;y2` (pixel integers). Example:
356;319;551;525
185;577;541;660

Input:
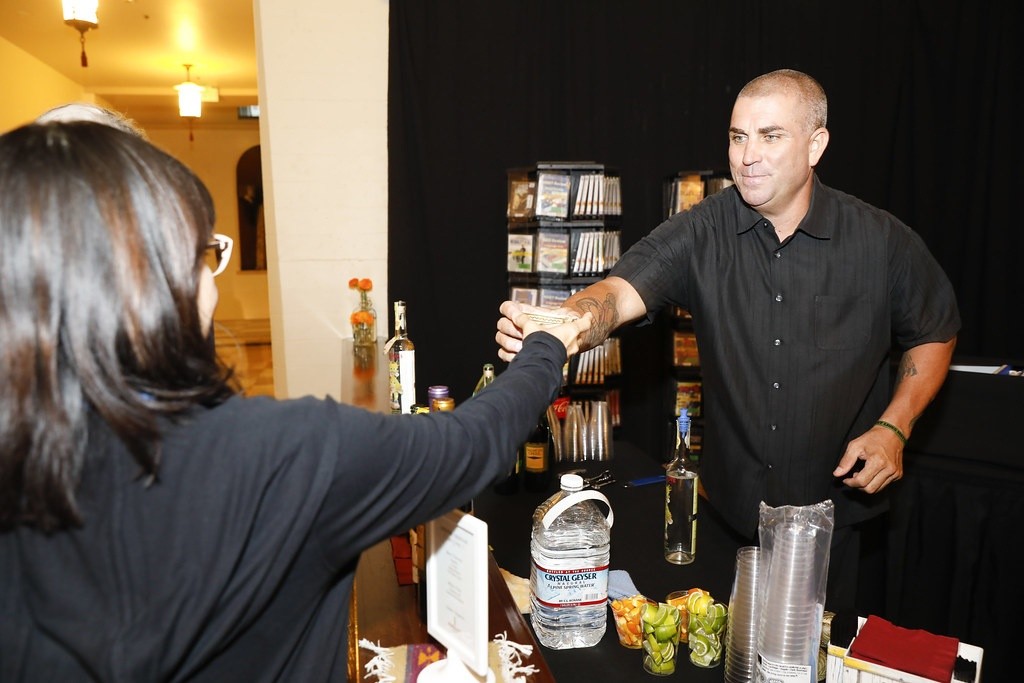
496;70;960;618
0;120;592;683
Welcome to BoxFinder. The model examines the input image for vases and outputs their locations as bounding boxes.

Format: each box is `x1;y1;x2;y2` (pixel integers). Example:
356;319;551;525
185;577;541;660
351;291;377;346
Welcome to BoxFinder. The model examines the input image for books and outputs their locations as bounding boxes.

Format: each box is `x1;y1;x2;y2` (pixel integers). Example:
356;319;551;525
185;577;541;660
505;175;621;429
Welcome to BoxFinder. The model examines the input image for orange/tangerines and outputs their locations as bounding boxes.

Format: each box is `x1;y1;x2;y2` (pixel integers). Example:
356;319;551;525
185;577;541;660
666;588;711;644
611;593;652;650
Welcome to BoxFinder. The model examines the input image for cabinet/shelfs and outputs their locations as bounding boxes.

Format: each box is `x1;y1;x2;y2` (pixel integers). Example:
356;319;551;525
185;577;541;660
504;158;628;483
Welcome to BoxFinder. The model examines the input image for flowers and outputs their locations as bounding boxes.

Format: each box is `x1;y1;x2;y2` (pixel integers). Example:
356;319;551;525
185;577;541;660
349;278;372;326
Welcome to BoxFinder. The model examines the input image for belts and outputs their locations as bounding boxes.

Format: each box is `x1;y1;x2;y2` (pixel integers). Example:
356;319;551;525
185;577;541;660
700;496;758;546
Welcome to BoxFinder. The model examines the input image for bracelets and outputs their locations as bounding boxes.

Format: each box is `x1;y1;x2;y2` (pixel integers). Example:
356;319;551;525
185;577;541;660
875;420;907;444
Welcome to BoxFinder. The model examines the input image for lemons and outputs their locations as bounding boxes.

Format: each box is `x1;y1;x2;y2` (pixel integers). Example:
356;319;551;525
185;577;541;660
640;603;681;677
686;591;727;668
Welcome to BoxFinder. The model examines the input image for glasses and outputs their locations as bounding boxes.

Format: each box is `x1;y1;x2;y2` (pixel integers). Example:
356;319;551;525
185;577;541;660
202;234;233;276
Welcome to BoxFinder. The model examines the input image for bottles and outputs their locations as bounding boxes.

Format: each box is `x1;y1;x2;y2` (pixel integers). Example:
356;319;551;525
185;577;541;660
494;448;521;493
389;301;416;414
664;409;698;565
523;416;550;477
432;398;454;412
529;473;613;650
473;364;497;398
428;386;449;413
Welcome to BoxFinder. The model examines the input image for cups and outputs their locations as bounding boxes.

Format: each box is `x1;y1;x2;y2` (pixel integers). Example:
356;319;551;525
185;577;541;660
546;406;563;462
724;523;816;683
610;595;657;649
641;606;683;676
590;401;613;460
563;405;589;461
665;591;690;644
686;601;728;668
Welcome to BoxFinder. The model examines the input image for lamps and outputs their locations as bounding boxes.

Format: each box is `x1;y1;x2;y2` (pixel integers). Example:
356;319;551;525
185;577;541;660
173;63;203;142
62;0;98;68
